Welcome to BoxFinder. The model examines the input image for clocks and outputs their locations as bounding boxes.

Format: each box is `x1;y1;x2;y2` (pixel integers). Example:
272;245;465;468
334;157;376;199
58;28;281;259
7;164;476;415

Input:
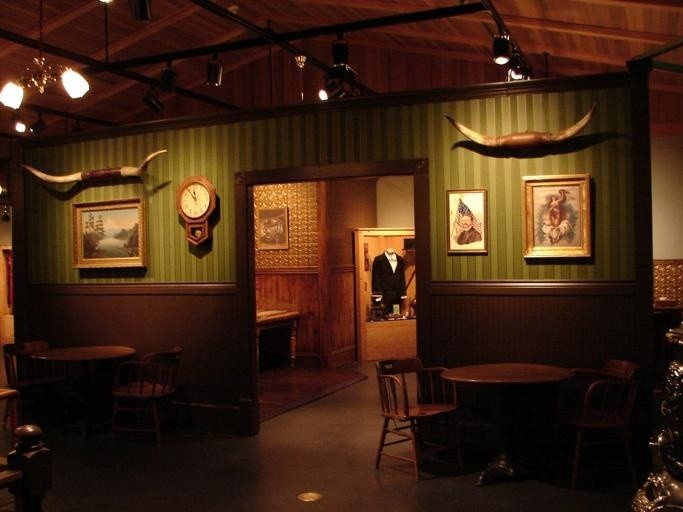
175;175;217;246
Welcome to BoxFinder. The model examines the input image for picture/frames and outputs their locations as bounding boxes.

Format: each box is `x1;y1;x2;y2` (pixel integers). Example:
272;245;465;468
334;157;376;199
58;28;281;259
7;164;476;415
256;206;289;251
445;188;488;255
71;197;148;269
520;173;592;259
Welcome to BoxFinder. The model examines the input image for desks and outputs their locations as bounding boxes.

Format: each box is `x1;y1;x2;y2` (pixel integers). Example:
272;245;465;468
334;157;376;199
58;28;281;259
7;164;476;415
256;310;301;372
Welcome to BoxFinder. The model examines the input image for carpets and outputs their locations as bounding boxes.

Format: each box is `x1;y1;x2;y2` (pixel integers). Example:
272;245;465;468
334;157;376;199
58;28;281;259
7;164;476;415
256;362;368;424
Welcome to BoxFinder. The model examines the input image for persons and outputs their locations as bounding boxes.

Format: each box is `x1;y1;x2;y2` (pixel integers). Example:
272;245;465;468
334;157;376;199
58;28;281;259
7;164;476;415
457;215;481;245
372;249;407;371
548;190;565;227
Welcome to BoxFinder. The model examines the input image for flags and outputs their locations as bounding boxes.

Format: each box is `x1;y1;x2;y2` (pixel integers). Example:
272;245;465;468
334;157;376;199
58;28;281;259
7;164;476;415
455;199;482;236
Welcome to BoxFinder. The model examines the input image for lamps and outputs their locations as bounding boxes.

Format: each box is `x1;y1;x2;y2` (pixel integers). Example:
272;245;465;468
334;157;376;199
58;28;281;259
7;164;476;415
317;34;358;102
128;0;153;21
489;30;528;84
204;57;223;88
0;0;92;111
143;92;162;113
29;112;47;136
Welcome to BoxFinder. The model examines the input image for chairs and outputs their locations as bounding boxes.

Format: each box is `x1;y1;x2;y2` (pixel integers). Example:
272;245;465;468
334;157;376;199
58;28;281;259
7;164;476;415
108;344;185;453
556;359;643;490
374;356;463;483
3;340;72;438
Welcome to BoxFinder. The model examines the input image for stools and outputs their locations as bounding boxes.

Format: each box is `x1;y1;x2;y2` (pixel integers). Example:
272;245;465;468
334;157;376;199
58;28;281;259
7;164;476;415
0;388;20;448
